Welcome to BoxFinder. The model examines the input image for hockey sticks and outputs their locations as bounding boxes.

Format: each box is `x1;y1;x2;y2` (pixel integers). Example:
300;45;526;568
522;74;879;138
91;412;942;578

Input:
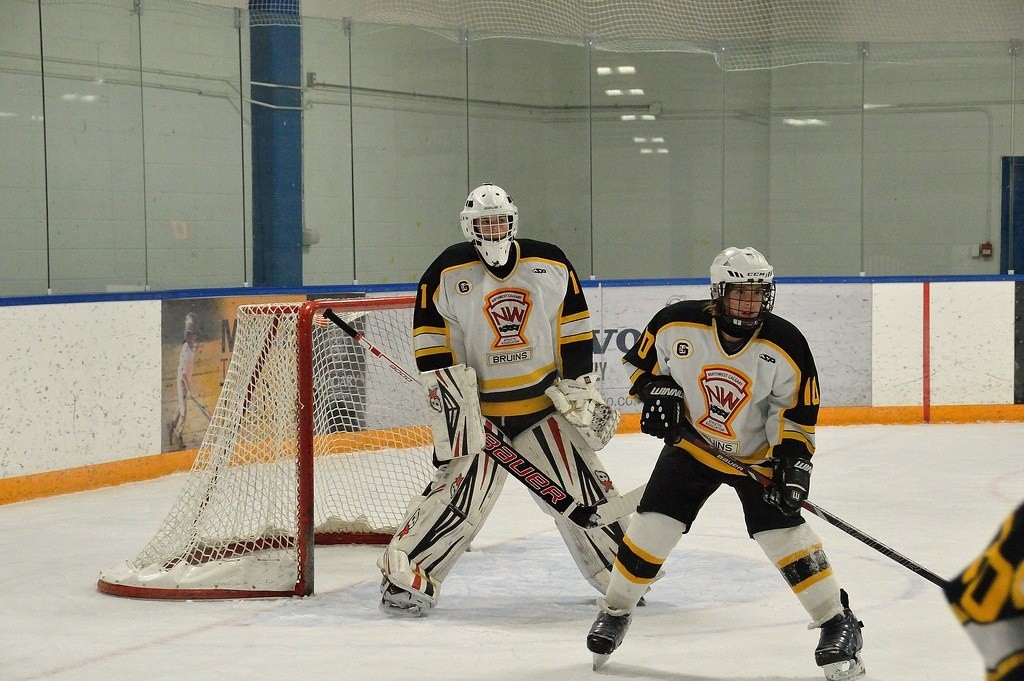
323;306;649;530
677;425;954;590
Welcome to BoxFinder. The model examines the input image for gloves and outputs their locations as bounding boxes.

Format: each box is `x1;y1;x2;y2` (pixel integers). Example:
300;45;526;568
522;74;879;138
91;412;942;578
762;454;813;517
639;374;685;449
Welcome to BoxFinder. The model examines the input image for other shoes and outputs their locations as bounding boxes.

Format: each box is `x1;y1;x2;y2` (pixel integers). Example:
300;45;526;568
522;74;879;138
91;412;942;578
380;575;429;618
173;433;185;448
167;423;173;445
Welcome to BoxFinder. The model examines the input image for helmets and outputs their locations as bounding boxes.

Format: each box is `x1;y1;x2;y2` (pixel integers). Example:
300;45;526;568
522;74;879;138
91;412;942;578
710;246;777;328
460;183;519;268
184;312;200;346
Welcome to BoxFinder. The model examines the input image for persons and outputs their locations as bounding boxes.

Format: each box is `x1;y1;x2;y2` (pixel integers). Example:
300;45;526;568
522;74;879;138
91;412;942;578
379;182;665;616
941;500;1024;681
166;312;197;447
585;246;869;681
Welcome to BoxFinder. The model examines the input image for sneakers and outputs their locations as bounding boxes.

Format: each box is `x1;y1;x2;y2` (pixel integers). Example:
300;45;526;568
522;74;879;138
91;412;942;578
586;606;632;671
814;588;864;681
